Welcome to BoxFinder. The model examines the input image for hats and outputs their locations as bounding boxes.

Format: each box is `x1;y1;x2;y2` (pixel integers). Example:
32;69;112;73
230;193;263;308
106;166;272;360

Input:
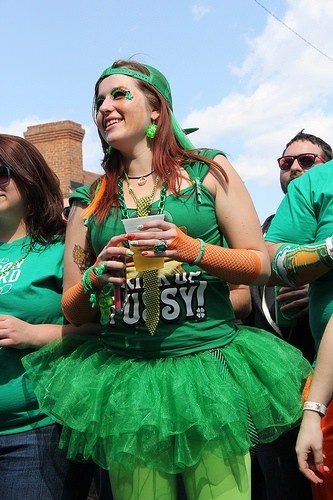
95;61;173;112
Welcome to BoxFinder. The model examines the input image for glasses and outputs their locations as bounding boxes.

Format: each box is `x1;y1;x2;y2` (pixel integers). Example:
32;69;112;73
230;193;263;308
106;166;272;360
0;163;12;187
277;152;327;170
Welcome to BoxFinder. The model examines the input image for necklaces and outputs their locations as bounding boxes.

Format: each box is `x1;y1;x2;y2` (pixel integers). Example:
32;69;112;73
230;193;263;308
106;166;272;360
0;238;34;275
123;168;157;186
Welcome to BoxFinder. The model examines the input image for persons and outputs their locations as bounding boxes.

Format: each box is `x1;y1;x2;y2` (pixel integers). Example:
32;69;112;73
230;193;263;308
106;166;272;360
227;128;333;499
0;133;113;500
20;60;314;500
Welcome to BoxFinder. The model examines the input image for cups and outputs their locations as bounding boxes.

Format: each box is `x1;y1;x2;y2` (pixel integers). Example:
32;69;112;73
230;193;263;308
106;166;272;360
121;214;165;271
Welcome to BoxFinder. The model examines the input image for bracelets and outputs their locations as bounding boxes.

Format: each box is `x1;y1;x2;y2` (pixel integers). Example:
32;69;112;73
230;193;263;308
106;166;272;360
303;401;326;415
190;238;205;266
81;266;113;325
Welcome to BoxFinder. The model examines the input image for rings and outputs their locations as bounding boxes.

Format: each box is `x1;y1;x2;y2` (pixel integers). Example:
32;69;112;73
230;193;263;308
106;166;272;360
100;260;107;272
153;239;168;254
